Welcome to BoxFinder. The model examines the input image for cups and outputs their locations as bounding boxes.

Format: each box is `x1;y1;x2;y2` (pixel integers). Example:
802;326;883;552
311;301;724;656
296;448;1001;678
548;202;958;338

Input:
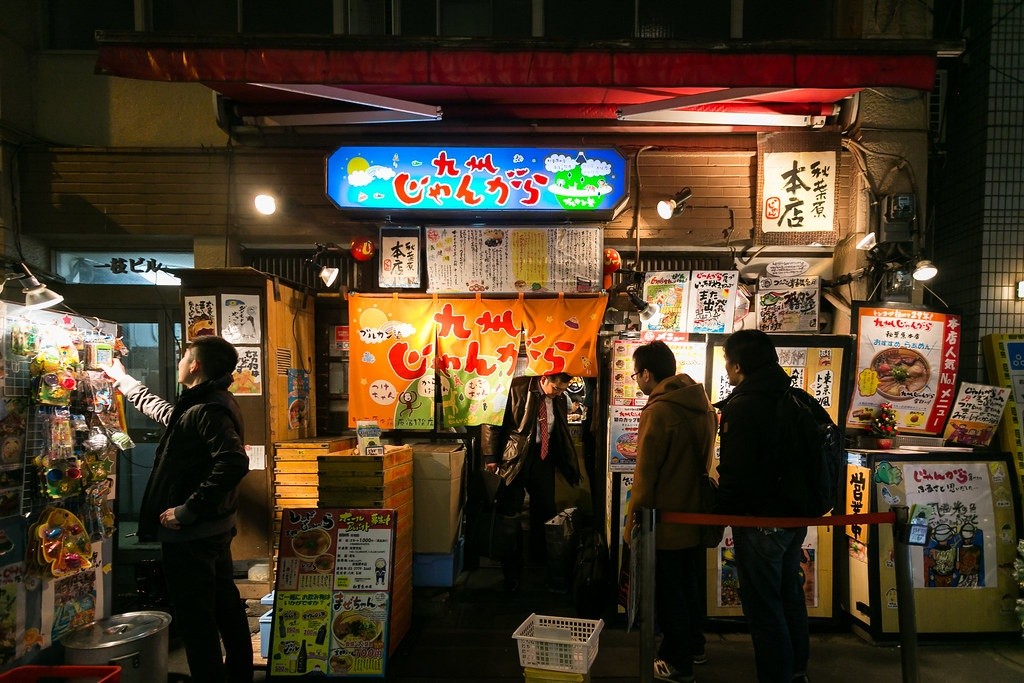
290;657;297;673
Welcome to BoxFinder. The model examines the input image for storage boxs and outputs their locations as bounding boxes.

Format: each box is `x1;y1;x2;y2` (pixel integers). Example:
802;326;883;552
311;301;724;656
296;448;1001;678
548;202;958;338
0;664;123;683
260;441;466;659
511;611;604;673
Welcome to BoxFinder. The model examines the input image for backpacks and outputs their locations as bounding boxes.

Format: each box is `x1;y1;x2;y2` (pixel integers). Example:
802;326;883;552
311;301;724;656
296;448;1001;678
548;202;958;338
780;378;845;519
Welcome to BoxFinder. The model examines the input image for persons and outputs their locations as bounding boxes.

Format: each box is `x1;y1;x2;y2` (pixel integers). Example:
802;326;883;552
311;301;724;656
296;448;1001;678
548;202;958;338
713;329;810;683
481;373;585;593
99;336;254;683
623;340;719;683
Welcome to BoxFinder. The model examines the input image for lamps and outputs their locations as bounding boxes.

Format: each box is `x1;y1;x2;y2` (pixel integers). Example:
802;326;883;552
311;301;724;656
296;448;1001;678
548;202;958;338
657;186;693;220
619;283;658;323
306;241;344;288
854;231;889;261
902;253;937;282
0;263;64;308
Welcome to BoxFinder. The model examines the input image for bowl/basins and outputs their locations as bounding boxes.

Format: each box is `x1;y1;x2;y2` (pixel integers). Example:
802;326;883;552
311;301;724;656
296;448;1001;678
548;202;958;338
313;554;335;574
332;609;382;652
871;347;932;401
329;654;354;673
292;528;331;562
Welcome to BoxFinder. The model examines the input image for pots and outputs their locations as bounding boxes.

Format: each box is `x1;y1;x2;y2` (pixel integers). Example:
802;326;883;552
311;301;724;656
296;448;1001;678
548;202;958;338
61;611;172;683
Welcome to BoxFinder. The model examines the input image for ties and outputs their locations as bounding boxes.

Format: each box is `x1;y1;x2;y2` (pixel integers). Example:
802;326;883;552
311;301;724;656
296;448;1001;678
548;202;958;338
537;394;548;461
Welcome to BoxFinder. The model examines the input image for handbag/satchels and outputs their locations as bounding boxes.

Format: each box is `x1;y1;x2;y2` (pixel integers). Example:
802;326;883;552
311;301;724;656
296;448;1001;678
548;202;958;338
467;470;504;506
700;476;727;549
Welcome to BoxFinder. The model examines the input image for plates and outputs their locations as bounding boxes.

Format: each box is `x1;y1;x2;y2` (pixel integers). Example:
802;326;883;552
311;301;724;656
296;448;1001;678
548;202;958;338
616;432;638;459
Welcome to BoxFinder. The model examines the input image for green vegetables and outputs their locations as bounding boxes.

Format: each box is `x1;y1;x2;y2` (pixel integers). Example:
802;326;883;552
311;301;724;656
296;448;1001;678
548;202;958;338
892;365;909;383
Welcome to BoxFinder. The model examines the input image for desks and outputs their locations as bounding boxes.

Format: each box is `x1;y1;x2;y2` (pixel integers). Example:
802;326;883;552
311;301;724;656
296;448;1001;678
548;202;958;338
117;521;162;610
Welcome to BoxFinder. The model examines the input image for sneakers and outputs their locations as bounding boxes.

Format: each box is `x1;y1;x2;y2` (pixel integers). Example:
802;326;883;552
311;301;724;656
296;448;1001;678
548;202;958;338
653;653;707;683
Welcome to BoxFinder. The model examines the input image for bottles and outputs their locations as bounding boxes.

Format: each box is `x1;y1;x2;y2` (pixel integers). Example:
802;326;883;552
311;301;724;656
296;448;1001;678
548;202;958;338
248;564;269;581
298;640;307;673
279;616;286;638
316;623;327;645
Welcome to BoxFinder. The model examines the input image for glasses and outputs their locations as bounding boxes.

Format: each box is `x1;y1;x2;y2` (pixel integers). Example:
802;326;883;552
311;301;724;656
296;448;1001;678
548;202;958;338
629;367;644;382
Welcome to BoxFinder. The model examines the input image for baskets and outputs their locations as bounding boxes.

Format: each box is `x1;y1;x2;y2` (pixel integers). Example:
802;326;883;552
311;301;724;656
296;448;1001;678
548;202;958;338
511;612;604;675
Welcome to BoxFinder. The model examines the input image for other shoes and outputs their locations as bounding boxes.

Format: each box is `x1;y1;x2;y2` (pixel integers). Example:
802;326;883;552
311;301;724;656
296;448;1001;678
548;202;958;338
529;551;553;562
505;576;520;593
